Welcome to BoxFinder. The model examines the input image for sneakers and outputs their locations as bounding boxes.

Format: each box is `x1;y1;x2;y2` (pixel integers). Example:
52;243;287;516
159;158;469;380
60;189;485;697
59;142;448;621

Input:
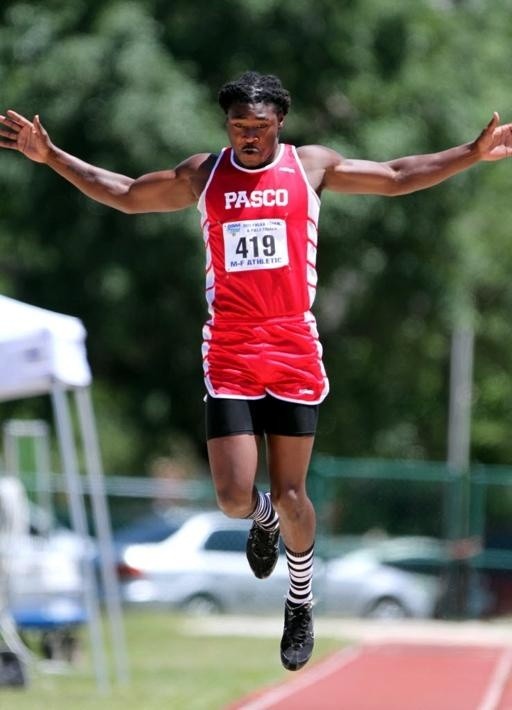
280;600;315;671
246;492;280;579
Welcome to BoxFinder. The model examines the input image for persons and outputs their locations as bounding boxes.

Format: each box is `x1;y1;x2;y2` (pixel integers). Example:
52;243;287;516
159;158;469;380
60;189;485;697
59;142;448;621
1;65;512;673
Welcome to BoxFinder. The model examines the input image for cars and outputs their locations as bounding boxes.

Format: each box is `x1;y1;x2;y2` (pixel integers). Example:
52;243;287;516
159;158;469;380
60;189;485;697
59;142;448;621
104;510;440;622
